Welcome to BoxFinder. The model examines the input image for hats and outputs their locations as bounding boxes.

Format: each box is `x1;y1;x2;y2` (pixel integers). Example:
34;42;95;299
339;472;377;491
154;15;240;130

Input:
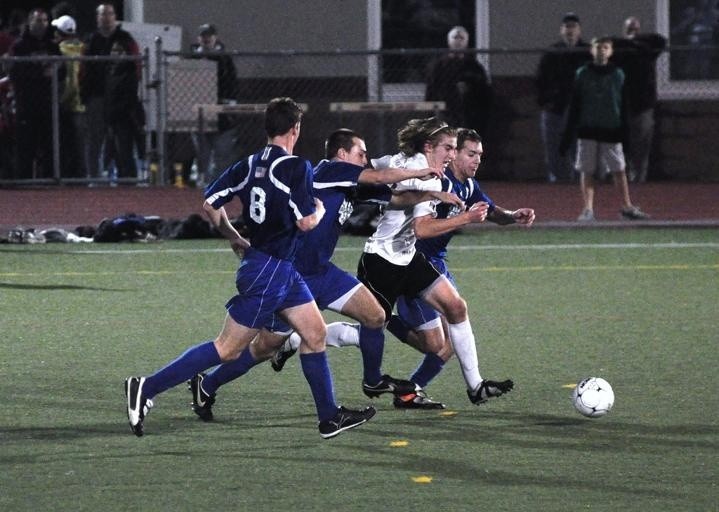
51;15;76;36
197;23;218;38
561;12;579;24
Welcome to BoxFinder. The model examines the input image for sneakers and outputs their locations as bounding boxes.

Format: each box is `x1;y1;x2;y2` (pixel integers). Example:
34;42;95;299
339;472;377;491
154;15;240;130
393;384;447;410
318;405;377;440
361;374;416;400
8;227;24;244
123;375;154;438
187;374;217;423
619;206;648;220
21;227;47;244
270;338;297;372
576;207;593;222
466;378;515;408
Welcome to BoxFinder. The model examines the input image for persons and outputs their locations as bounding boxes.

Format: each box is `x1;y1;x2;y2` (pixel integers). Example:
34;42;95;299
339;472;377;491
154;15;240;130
325;126;537;417
122;96;375;441
426;26;491;136
534;13;594;185
611;17;665;184
270;117;514;405
0;0;157;191
562;37;649;220
182;24;238;189
187;126;417;421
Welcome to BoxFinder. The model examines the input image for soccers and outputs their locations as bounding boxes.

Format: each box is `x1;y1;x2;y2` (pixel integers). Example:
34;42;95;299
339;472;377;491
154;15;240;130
574;378;614;418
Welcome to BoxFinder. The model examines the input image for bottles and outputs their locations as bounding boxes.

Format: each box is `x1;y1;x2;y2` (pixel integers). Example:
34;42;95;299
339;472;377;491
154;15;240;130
109;154;199;188
